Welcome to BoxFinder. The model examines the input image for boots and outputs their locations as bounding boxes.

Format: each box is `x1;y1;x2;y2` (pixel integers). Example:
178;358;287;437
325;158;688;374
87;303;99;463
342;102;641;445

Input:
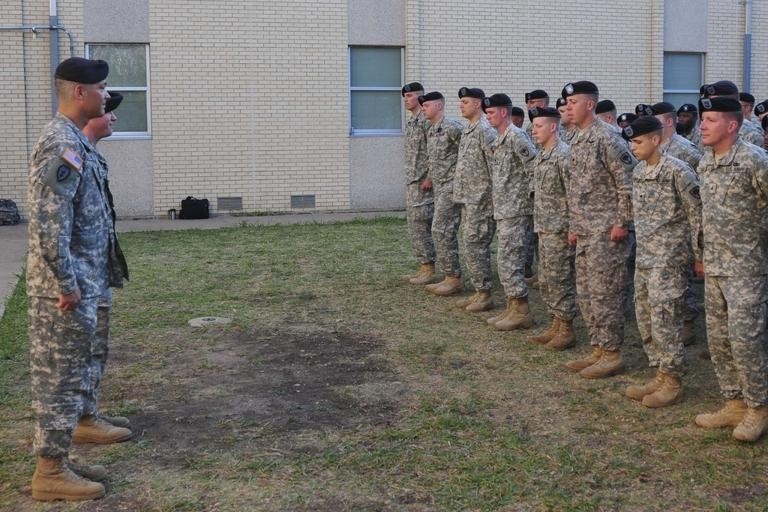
408;263;464;296
71;414;134;444
627;374;684;407
457;290;534;331
526;318;625;378
695;400;767;441
30;455;105;500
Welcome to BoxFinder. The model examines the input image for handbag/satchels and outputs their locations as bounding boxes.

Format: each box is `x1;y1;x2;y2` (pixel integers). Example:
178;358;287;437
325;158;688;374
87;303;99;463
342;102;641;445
178;197;209;219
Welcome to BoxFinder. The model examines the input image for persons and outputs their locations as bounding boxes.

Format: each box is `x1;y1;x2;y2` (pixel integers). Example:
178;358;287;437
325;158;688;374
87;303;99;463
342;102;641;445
452;87;497;312
25;56;111;502
482;80;636;379
402;82;436;285
70;92;134;445
596;80;766;443
417;91;466;297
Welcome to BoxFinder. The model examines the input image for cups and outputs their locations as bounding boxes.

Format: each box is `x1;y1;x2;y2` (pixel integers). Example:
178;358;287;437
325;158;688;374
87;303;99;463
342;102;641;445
168;209;176;220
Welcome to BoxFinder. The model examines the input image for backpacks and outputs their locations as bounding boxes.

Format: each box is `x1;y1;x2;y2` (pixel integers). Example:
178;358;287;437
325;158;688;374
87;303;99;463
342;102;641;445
0;199;21;224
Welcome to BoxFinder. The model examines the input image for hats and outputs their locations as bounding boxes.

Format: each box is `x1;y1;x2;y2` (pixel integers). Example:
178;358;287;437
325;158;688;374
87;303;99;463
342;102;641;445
56;56;109;84
616;103;697;140
458;82;614;121
401;83;423;97
418;92;444;107
104;93;124;112
700;81;768;131
